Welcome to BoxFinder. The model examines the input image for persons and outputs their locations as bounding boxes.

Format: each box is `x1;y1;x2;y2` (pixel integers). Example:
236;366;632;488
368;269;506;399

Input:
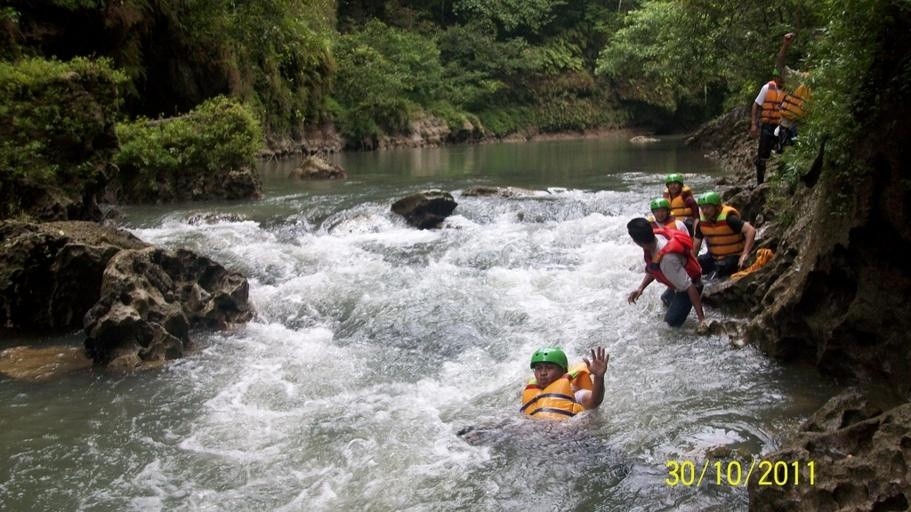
645;198;689;245
625;216;711;337
660;173;700;241
521;344;610;420
773;29;817;154
688;190;757;281
750;69;785;186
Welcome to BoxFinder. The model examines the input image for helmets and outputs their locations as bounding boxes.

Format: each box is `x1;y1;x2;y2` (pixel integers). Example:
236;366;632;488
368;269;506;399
773;66;790;77
697;192;722;208
666;174;684;186
650;198;670;212
530;346;569;371
